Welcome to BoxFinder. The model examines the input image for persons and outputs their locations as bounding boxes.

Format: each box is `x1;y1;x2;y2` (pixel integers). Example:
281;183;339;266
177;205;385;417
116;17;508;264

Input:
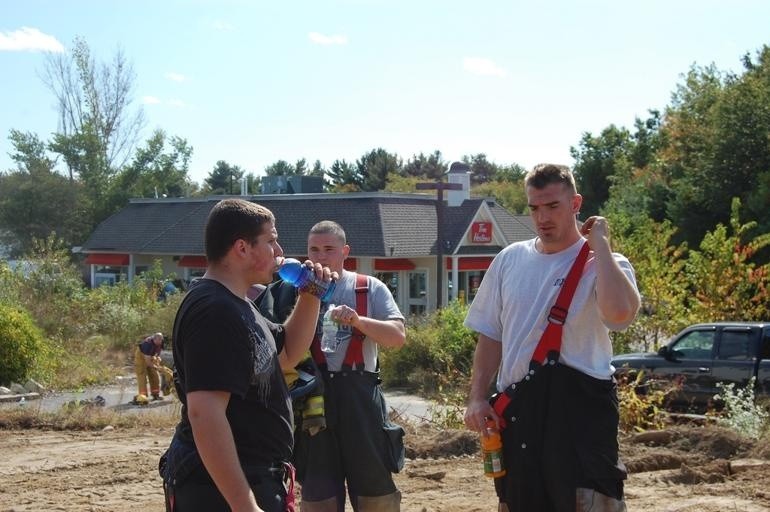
462;166;641;511
254;220;408;512
134;333;165;399
160;199;339;512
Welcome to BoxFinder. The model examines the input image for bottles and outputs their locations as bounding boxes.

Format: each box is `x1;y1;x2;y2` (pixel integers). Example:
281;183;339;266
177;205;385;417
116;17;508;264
321;303;339;353
478;418;508;479
276;257;339;302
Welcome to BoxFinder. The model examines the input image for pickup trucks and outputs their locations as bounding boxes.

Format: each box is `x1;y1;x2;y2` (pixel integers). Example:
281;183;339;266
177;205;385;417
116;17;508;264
608;321;769;423
